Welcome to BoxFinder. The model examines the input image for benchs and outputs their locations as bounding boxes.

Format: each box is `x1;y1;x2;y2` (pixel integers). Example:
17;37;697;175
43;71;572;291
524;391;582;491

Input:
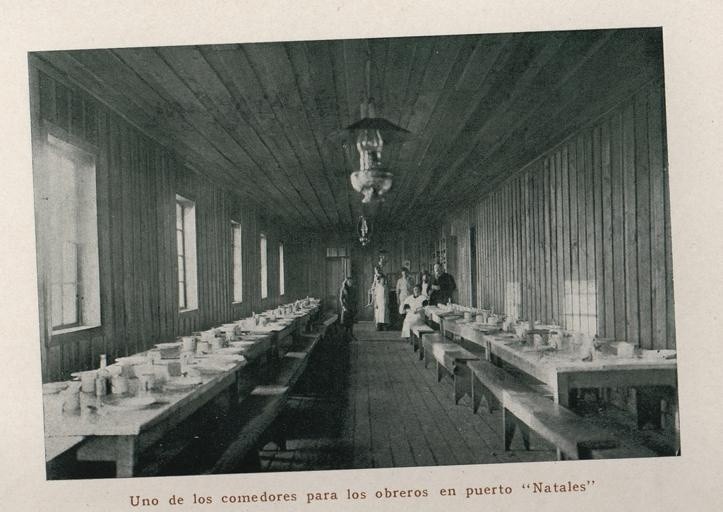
411;302;678;460
40;296;338;480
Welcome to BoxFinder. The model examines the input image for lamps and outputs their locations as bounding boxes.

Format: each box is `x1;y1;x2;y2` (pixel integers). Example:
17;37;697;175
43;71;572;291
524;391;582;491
339;52;410;204
357;213;373;246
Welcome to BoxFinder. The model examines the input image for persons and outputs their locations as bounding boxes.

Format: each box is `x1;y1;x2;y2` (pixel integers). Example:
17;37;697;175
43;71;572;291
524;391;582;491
364;262;456;339
337;277;358;337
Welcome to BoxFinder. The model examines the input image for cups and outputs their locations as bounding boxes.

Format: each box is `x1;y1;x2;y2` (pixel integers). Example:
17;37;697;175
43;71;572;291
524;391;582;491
423;299;676;369
43;295;322;423
